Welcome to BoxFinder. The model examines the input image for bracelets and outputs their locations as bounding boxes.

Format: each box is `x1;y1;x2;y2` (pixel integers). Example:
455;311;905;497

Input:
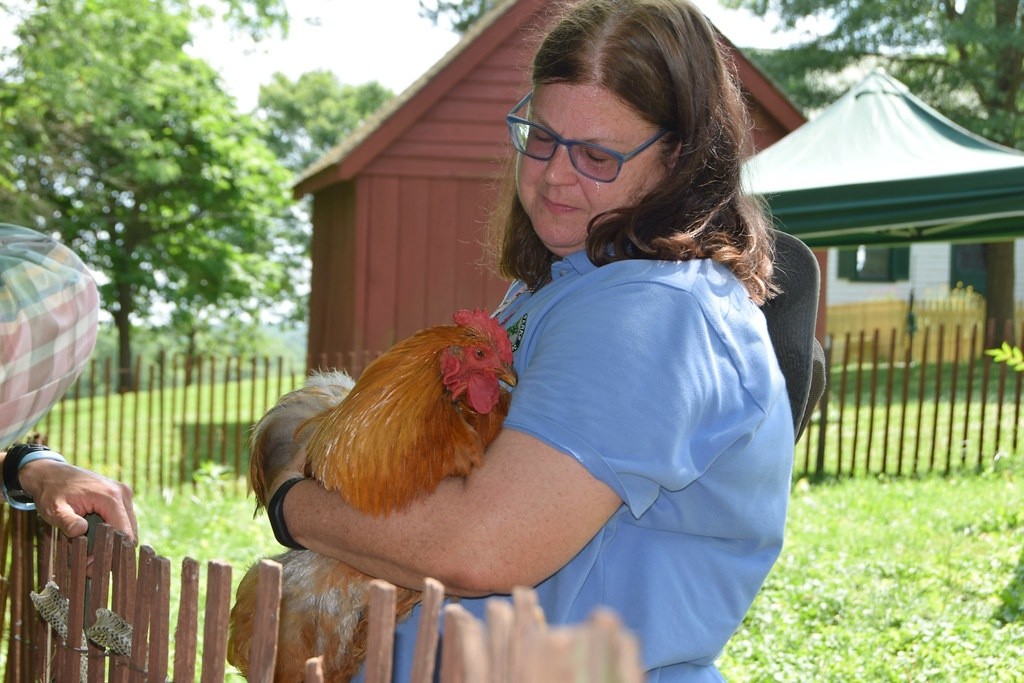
0;449;66;512
2;441;51;505
267;475;310;552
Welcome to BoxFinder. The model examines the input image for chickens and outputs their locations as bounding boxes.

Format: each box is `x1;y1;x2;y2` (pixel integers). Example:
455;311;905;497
223;306;522;683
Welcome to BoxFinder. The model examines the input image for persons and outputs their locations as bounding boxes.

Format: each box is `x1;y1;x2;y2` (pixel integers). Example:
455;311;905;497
262;0;793;683
1;219;141;552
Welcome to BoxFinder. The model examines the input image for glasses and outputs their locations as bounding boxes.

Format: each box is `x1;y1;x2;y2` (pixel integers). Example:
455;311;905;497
506;89;671;184
